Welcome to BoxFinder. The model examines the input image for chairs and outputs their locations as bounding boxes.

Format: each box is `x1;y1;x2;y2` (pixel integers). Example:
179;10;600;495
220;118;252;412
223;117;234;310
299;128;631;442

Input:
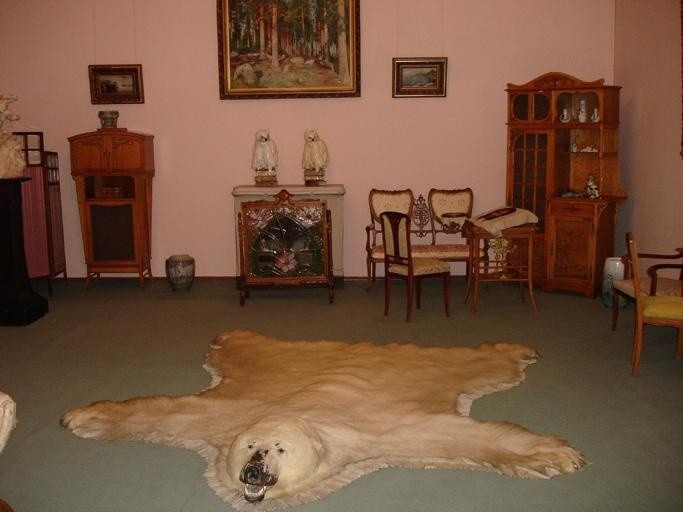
608;234;681;343
625;232;682;380
378;209;452;323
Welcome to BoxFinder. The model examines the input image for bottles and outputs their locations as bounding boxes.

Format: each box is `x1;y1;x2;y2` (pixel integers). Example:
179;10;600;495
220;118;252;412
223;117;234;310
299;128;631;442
575;135;581;151
578;100;586;123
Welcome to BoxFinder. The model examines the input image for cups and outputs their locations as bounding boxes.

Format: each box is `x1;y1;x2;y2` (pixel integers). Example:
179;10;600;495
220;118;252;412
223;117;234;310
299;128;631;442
571;143;577;152
589;186;598;196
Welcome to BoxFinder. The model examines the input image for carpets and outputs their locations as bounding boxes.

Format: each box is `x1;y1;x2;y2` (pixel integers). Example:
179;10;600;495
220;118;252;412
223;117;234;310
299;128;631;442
59;328;592;511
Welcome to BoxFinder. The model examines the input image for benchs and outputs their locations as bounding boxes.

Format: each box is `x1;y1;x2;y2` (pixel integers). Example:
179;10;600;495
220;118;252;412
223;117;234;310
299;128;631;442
365;187;492;291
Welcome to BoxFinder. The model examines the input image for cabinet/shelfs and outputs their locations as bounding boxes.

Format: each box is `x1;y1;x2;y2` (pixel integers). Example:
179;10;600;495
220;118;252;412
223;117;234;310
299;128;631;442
65;109;157;288
501;70;629;300
231;181;346;306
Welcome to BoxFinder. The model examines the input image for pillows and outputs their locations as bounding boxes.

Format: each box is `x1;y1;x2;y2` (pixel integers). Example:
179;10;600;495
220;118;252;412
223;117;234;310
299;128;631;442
463;207;537;241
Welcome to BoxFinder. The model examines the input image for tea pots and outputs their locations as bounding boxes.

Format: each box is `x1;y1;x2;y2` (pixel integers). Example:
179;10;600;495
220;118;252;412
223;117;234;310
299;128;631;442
584;175;594;194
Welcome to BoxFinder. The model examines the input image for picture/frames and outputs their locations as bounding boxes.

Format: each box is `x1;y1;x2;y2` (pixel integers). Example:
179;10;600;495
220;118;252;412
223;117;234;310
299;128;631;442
87;63;144;105
390;56;447;101
213;1;361;101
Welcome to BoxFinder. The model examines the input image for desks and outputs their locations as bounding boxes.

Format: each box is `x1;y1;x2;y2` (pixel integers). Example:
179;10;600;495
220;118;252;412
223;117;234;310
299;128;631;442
463;218;543;317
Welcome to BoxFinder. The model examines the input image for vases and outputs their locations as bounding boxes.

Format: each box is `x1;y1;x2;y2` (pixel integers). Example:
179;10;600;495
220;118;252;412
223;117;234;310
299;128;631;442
599;256;629;309
163;253;195;295
